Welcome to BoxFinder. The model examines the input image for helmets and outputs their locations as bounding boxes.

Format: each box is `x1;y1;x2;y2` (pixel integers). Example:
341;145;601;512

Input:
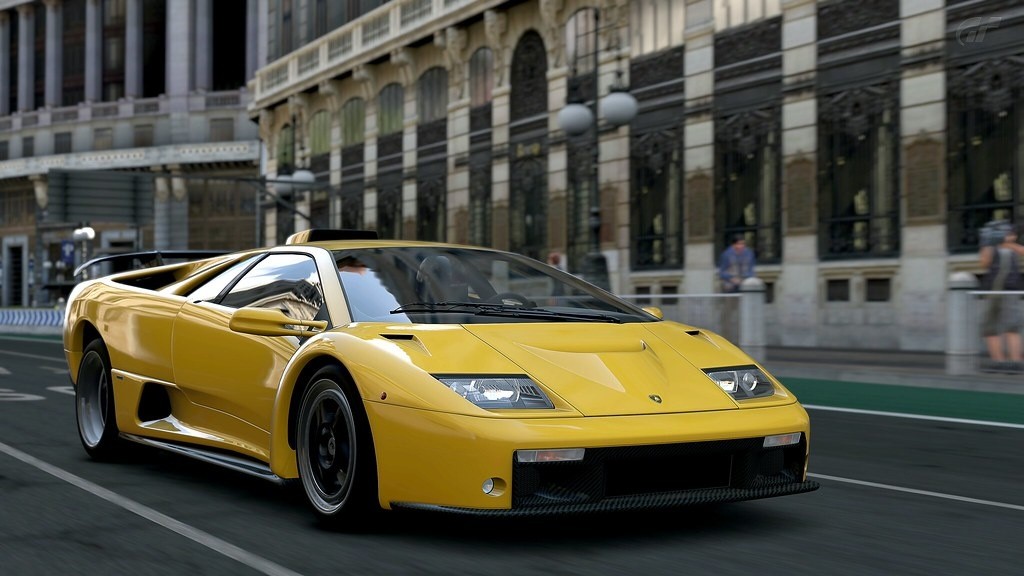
415;253;470;304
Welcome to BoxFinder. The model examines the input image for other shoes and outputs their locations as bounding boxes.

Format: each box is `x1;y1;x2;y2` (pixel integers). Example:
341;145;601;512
979;360;1024;374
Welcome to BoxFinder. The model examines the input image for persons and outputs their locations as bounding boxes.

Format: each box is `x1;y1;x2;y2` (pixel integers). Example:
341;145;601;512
416;254;469;309
977;222;1024;368
546;253;566;297
717;234;757;340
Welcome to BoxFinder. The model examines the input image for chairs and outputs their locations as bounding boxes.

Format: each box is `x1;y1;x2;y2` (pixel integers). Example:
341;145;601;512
413;256;479;322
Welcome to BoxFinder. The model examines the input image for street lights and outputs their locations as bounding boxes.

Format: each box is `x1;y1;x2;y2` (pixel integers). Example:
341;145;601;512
71;227;96;281
556;92;639;305
273;169;314;233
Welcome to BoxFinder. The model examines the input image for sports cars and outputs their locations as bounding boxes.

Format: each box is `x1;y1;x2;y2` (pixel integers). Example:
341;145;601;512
59;225;821;532
